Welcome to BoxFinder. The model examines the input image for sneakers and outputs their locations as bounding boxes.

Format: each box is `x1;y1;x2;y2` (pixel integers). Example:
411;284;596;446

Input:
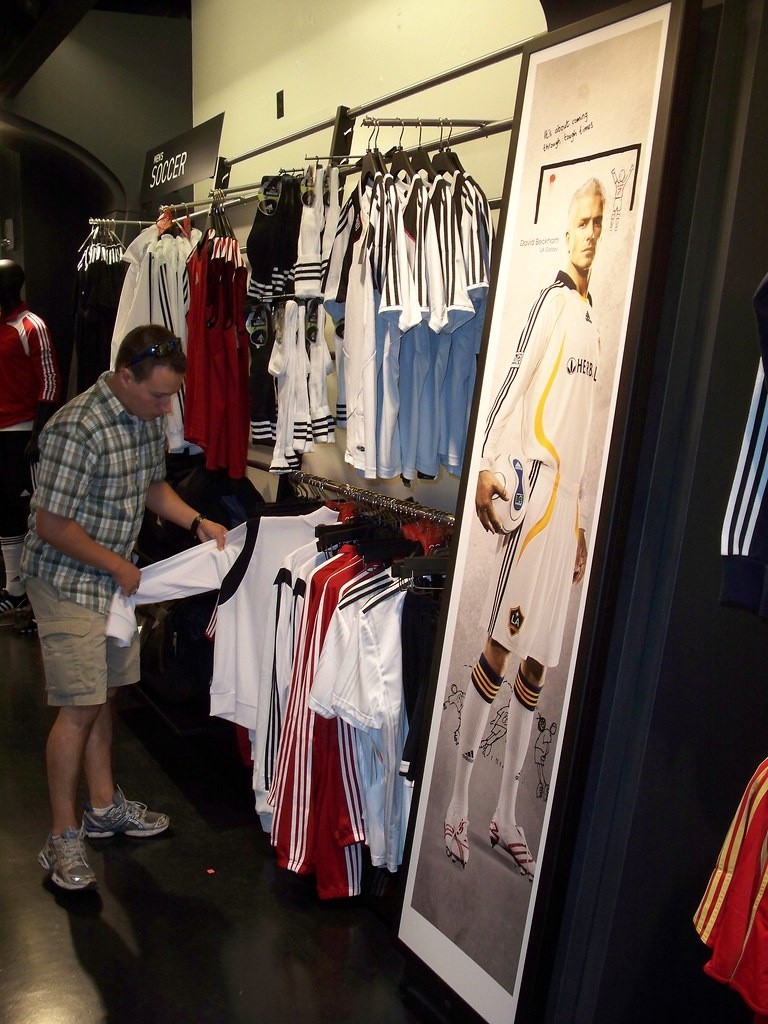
0;588;31;616
443;809;470;869
13;610;38;632
37;826;97;890
82;784;170;837
488;808;535;881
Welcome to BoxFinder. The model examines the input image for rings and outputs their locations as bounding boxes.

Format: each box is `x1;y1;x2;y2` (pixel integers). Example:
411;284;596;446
480;509;485;513
132;590;137;595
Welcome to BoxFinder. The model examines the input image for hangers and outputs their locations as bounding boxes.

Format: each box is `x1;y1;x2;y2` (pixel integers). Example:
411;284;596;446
410;117;438;182
389;117;417;182
445;118;466;176
427;116;456;178
256;467;456;598
75;115;390;329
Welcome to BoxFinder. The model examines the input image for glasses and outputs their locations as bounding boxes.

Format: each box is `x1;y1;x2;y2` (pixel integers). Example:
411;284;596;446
128;337;182;369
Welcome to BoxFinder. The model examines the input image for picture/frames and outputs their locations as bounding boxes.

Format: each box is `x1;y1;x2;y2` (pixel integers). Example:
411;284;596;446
389;0;701;1024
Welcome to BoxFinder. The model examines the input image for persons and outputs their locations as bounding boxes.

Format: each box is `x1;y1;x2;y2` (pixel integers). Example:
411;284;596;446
18;324;229;892
0;259;57;634
443;177;603;881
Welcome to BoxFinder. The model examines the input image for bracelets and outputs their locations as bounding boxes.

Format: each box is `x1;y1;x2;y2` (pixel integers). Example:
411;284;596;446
190;514;205;538
